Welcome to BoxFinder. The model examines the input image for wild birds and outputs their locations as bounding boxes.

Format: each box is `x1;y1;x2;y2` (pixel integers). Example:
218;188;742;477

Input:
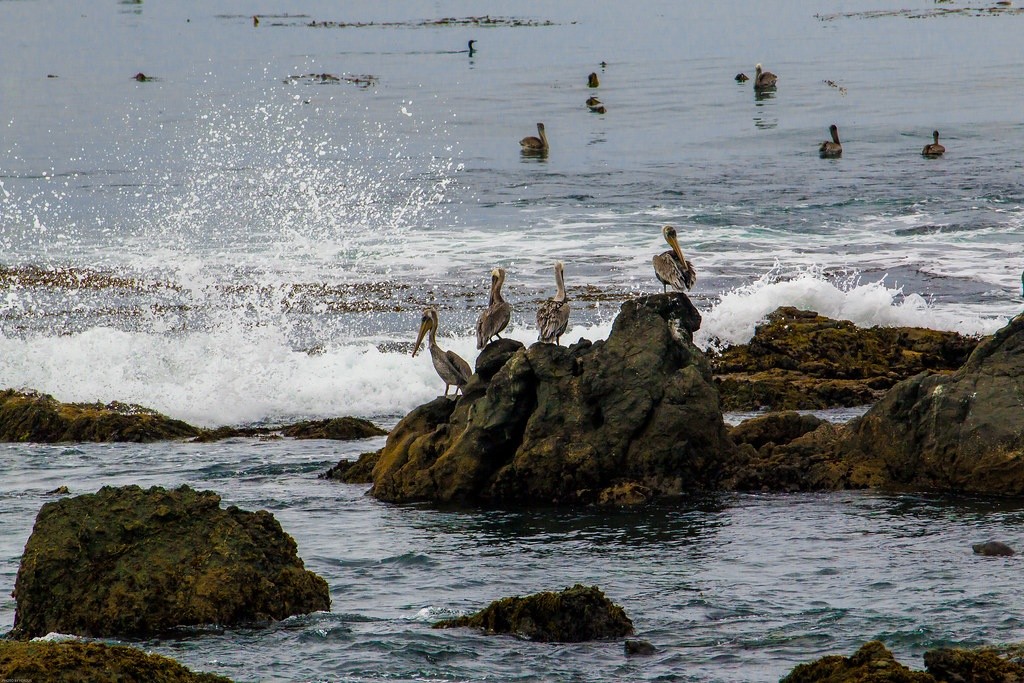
412;308;472;397
652;225;697;295
476;268;510;350
467;40;479;50
754;63;778;89
921;130;945;155
519;122;549;151
534;262;571;347
819;125;842;156
